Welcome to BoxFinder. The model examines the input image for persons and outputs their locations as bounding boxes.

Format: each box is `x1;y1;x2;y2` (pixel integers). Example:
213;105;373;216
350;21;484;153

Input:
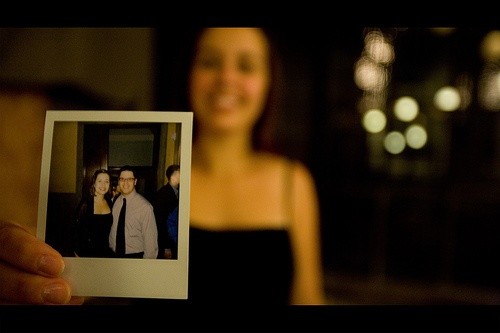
151;164;180;260
106;165;159;260
67;167;117;259
0;23;330;309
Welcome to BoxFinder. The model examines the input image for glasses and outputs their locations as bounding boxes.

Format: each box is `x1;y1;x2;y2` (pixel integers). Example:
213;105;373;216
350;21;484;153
118;177;135;182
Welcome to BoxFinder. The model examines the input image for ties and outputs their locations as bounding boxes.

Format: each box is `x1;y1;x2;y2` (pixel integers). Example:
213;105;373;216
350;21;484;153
116;198;126;256
